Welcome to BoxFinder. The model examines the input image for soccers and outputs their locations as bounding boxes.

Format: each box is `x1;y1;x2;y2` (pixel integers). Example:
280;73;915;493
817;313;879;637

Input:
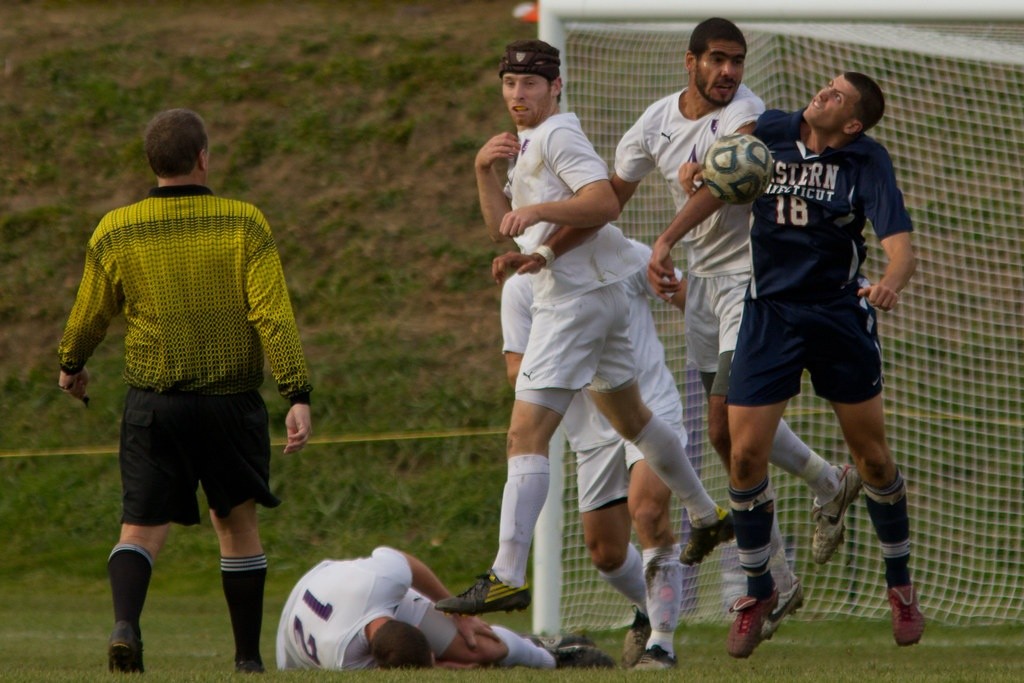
702;133;773;205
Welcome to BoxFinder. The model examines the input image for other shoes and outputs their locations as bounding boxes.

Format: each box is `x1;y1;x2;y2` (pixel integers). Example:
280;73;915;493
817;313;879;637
236;661;262;674
108;620;143;673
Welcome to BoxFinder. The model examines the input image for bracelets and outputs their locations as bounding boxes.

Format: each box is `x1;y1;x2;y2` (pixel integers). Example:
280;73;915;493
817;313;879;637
532;244;555;267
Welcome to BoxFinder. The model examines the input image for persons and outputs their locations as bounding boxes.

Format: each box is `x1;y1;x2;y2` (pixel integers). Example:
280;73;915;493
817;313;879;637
647;73;925;657
56;109;311;674
276;547;613;673
434;42;734;618
494;233;691;673
491;17;864;639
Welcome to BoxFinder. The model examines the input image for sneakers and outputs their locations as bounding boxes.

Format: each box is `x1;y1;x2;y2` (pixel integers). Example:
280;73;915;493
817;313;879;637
621;606;653;668
522;632;593;651
548;644;616;671
636;646;678;673
679;506;734;565
887;581;924;647
728;576;779;659
435;569;530;616
810;464;863;565
